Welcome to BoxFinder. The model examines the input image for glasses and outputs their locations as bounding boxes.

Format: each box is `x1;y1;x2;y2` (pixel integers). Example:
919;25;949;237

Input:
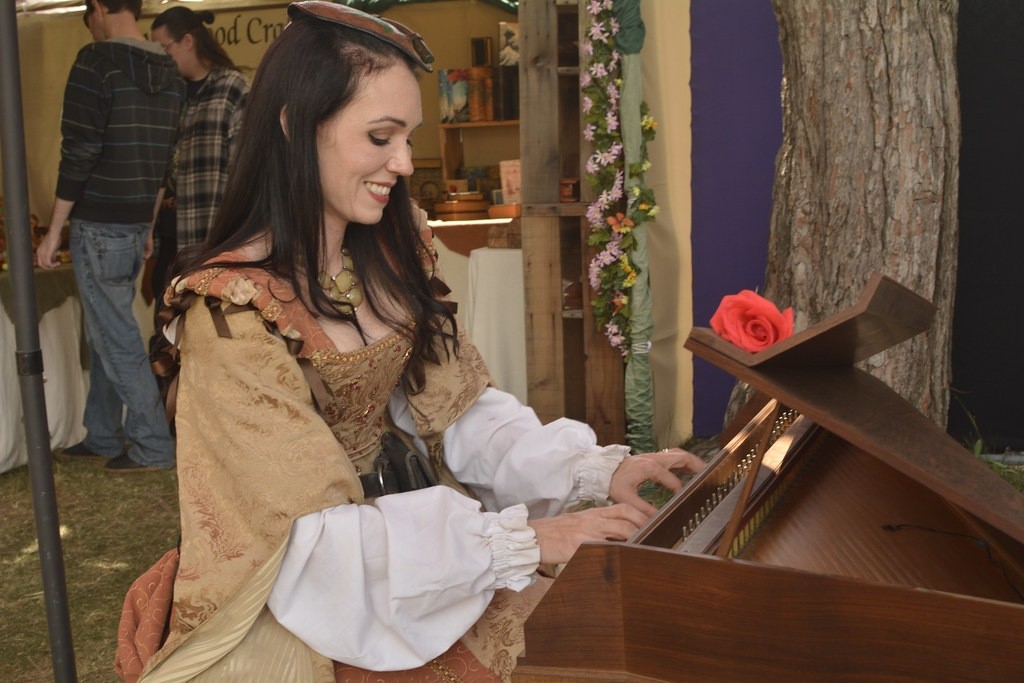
83;5;94;28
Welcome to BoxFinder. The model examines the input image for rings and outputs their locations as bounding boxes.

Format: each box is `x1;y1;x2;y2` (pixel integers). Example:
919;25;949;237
662;448;669;453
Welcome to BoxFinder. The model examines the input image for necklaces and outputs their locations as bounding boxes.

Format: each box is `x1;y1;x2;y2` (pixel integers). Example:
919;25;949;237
290;247;365;314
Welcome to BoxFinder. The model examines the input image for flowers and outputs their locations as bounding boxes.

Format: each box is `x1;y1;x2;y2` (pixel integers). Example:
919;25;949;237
710;286;795;353
580;0;659;363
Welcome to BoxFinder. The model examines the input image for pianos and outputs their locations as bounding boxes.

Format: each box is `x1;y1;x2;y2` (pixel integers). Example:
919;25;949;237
508;269;1024;682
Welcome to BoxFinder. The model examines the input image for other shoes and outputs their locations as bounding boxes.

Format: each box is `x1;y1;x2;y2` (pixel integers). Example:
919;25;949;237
105;455;158;471
58;442;100;458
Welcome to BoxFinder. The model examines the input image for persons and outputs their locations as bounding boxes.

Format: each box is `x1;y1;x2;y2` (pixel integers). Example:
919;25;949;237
112;0;707;683
36;0;252;473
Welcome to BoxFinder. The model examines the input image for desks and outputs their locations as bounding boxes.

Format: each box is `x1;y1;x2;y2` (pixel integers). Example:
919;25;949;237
0;257;158;474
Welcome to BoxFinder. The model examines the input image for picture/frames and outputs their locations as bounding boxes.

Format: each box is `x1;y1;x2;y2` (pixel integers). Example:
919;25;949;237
404;158;443;220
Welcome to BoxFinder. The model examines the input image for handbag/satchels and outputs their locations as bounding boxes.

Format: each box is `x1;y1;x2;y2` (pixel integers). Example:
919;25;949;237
381;432;439;493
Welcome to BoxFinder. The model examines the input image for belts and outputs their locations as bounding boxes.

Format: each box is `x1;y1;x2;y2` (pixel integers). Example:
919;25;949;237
358;456;398;500
